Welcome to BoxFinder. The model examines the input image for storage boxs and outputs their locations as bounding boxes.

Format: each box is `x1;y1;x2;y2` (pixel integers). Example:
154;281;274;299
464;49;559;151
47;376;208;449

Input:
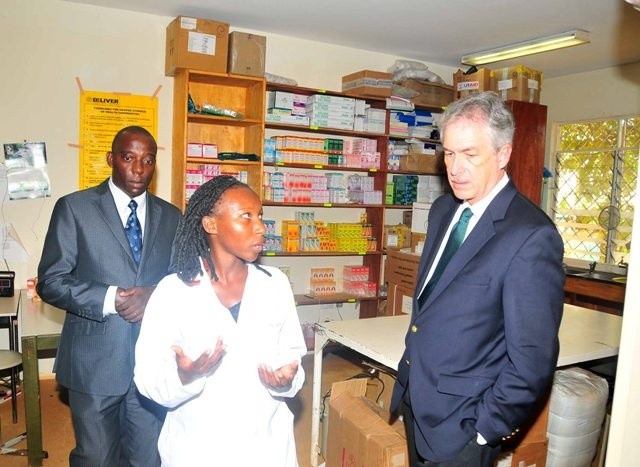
489;64;542;103
326;371;410;467
494;439;548;467
165;15;229;76
384;247;428;291
514;399;551;444
394;78;453;106
453;68;490;102
385;283;414;316
342;70;393;97
227;30;266;77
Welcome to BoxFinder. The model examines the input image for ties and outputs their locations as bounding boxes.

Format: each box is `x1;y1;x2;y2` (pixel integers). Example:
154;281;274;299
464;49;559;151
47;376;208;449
124;200;143;270
418;207;474;312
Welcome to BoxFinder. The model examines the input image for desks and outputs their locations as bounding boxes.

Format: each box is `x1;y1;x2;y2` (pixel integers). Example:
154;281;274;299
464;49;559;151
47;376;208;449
19;287;66;467
310;302;623;467
0;289;19;384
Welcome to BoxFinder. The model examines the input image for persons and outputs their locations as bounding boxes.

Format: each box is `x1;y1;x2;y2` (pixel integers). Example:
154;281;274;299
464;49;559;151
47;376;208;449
35;127;182;465
389;89;567;466
133;174;308;467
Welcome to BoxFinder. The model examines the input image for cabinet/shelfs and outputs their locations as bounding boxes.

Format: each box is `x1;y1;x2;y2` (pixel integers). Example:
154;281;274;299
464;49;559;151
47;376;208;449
171;67;266;216
258;81;549;353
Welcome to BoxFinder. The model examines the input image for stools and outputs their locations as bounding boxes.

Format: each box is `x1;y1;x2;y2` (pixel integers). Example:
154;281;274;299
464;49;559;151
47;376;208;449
0;349;33;426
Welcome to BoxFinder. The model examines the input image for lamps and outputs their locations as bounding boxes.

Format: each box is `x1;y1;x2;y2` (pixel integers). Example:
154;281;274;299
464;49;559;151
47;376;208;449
462;30;591;67
